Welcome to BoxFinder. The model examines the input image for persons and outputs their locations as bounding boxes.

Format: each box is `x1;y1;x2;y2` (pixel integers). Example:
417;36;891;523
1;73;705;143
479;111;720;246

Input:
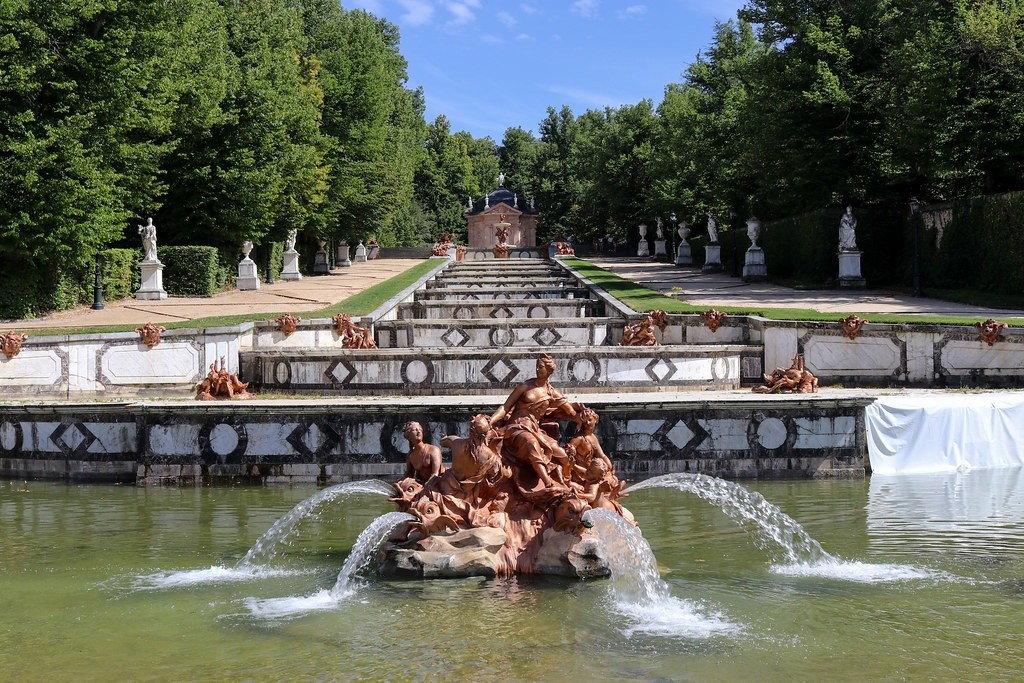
394;353;618;530
839;206;857;251
557;242;574;254
655;216;664;239
195;357;249;400
752;356;820;392
138;217;159;263
286;228;297;251
706;211;718;242
495;226;509;249
342;321;369;349
624;316;656;346
433;231;455;255
497;172;504;186
